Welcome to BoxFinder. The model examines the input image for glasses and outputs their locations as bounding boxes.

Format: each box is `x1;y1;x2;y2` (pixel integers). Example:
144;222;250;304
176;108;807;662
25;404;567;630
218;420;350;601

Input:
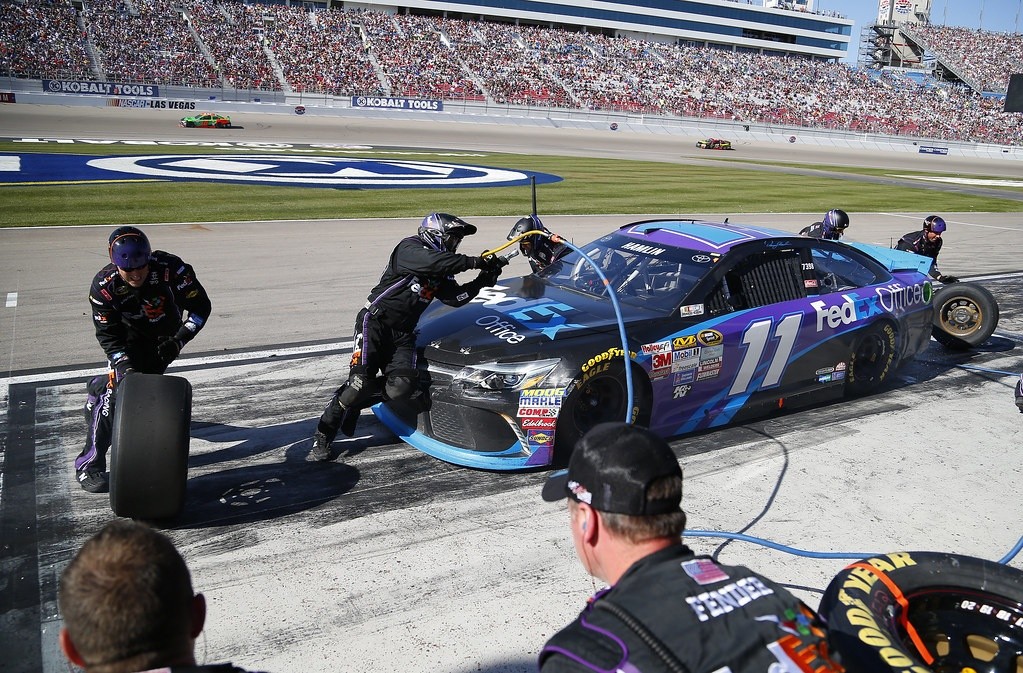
442;231;464;252
120;263;148;272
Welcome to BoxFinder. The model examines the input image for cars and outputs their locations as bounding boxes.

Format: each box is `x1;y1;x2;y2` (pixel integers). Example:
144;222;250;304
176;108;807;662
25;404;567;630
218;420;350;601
179;112;231;128
370;219;935;472
696;138;731;150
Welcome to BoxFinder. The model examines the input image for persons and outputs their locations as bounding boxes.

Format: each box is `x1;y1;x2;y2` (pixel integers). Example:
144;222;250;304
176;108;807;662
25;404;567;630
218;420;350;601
0;0;619;112
612;34;1023;146
822;9;825;16
312;212;502;463
537;422;850;673
798;208;849;240
75;227;212;492
828;9;831;16
894;216;959;284
507;214;574;273
833;10;836;17
899;21;1023;57
58;521;271;673
838;10;840;18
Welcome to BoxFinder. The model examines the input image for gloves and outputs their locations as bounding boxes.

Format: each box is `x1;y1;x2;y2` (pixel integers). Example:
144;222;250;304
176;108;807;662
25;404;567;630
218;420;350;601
939;275;959;285
157;325;194;365
477;268;502;287
476;249;497;270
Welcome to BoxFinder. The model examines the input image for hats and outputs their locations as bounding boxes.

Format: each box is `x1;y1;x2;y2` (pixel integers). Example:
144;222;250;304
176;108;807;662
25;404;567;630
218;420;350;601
542;422;683;515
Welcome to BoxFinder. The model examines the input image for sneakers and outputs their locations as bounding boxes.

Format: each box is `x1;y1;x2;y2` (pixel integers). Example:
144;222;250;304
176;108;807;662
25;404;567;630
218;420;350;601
84;376;100;425
312;429;333;460
341;407;360;436
76;468;108;492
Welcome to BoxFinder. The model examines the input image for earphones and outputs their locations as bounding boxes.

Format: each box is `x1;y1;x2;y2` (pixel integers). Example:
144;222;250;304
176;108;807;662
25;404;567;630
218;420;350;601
582;521;587;531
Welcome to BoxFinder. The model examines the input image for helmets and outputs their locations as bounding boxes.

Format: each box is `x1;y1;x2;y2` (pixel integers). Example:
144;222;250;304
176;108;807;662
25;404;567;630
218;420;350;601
419;212;477;252
824;209;849;233
923;215;946;233
108;226;151;268
506;217;534;256
